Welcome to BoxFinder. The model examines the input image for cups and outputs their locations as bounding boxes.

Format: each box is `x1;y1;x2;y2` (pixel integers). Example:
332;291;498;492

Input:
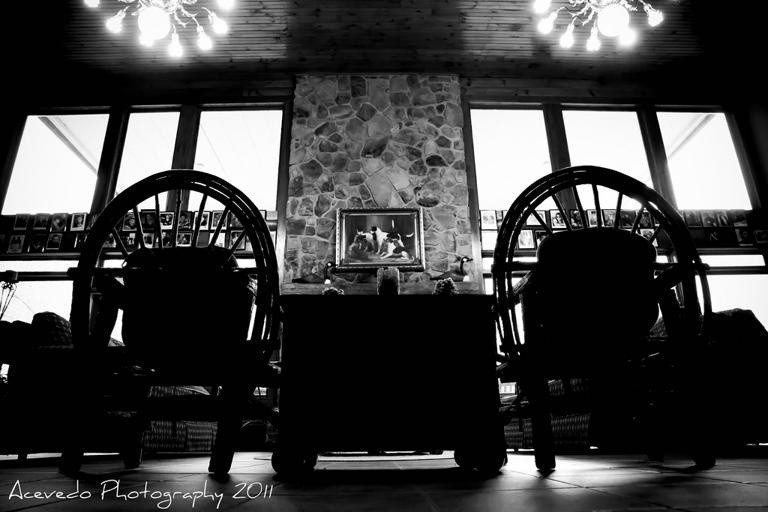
376;267;400;295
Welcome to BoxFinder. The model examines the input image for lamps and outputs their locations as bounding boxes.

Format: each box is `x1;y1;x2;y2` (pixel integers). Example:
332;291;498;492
533;1;666;56
84;0;235;59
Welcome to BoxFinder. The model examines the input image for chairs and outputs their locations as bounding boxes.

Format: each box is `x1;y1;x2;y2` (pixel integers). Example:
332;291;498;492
57;167;281;477
490;162;719;475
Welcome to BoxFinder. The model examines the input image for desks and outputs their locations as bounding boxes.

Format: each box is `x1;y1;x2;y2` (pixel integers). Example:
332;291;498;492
271;292;508;490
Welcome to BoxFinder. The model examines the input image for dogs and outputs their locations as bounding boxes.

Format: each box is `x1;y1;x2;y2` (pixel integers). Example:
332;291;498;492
353;219;415;262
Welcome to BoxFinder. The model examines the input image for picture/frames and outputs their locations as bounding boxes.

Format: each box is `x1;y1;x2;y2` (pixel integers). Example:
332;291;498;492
478;208;768;257
5;208;267;254
336;205;426;274
